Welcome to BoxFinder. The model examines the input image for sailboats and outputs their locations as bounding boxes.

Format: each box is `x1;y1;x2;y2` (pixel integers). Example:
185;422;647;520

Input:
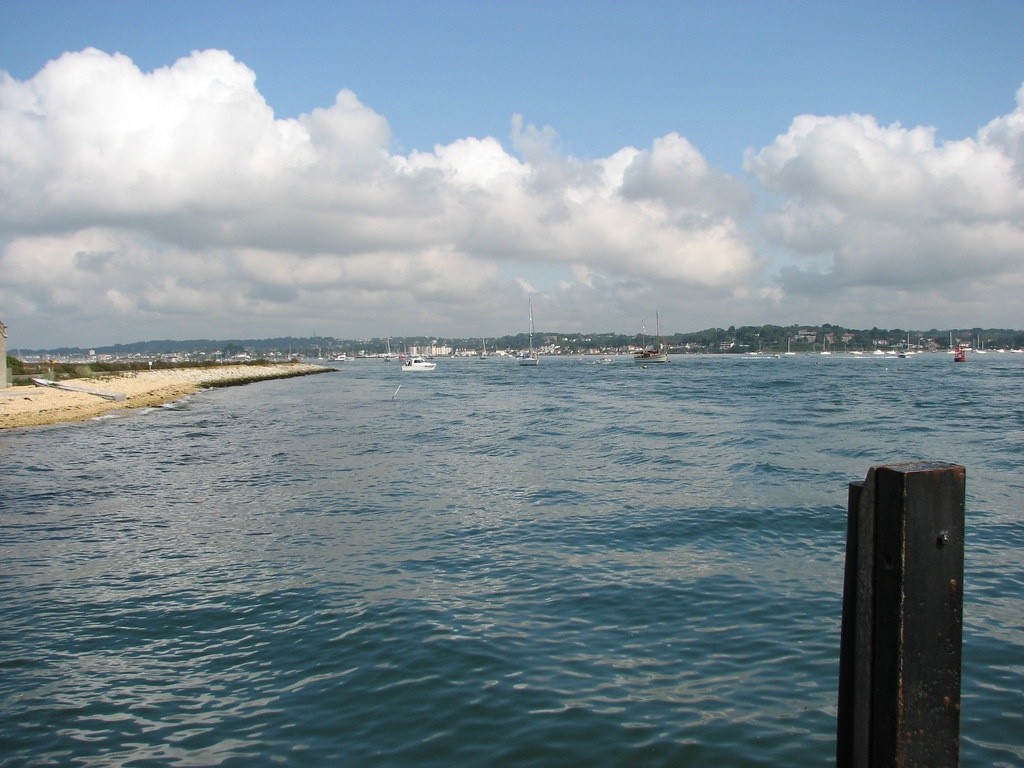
947;331;957;355
819;336;832;356
785;337;796;356
517;296;541;367
632;310;668;363
740;341;780;360
974;334;1023;354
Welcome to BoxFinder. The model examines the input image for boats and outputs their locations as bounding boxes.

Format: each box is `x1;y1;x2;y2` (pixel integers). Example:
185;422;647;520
954;348;968;362
592;356;613;365
850;334;924;357
401;354;438;372
230;342;399;365
